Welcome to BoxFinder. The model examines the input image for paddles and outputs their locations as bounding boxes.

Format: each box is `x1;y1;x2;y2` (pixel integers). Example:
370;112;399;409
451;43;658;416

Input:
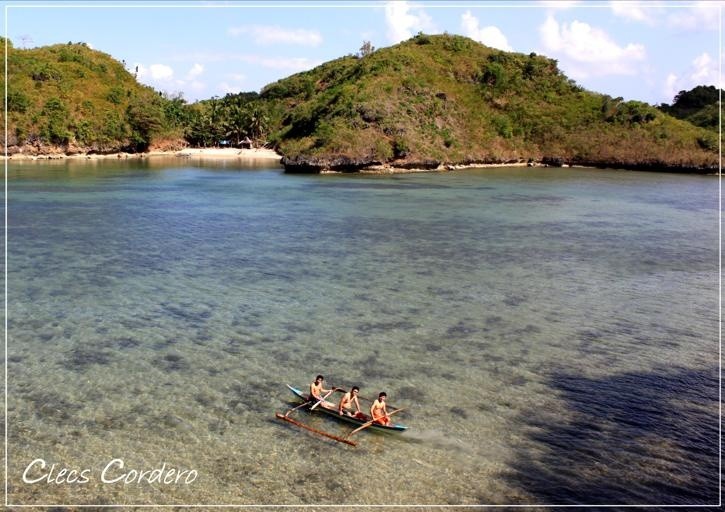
350;407;407;436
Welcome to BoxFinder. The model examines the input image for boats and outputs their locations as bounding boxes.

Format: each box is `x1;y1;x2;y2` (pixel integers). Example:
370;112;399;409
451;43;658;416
283;381;412;433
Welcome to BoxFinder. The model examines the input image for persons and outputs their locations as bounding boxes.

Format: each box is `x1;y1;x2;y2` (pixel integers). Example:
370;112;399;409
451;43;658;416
369;391;391;426
309;374;339;410
337;384;361;420
